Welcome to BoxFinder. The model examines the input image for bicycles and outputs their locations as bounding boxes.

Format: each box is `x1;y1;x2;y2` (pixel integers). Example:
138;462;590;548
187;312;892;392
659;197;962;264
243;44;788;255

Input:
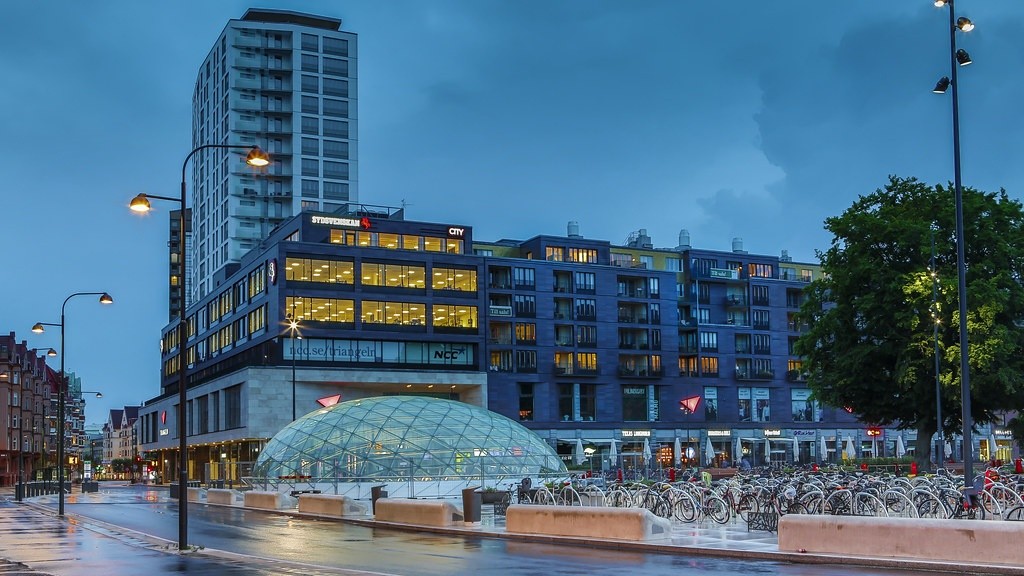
501;466;1024;535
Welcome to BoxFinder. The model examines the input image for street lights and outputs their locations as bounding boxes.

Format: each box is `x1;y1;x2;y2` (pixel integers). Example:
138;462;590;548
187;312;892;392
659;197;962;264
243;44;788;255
0;347;57;501
129;144;270;550
57;392;104;481
932;0;976;488
291;319;297;422
31;291;115;515
